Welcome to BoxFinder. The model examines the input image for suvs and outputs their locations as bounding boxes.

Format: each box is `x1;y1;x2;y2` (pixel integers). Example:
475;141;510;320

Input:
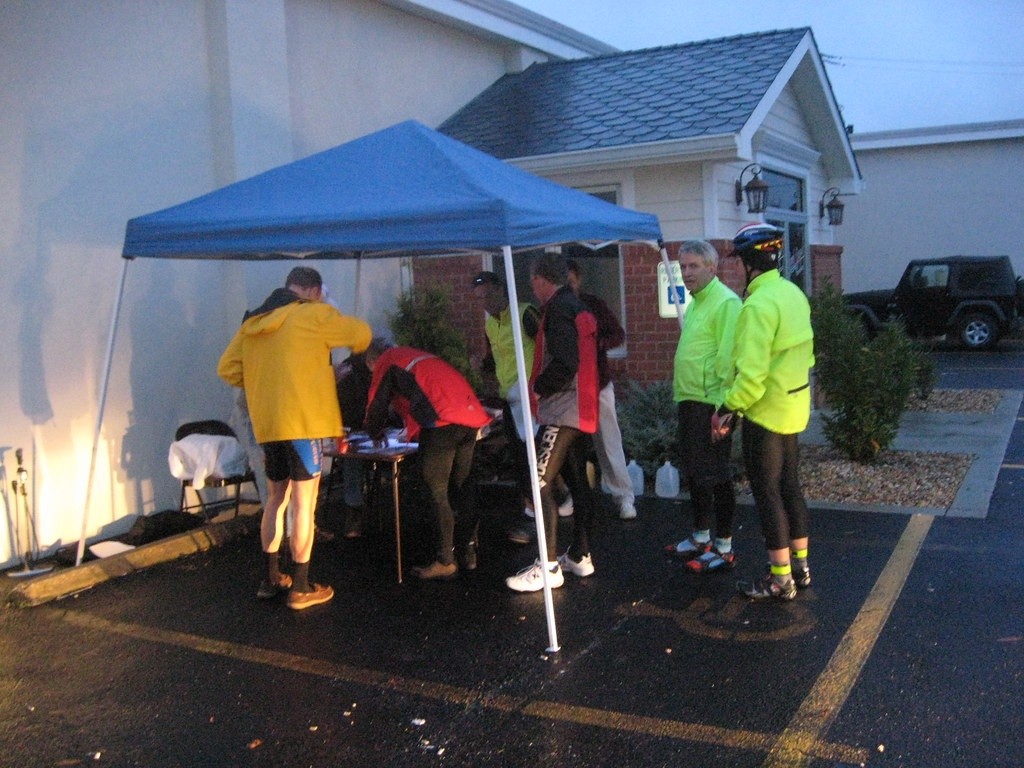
844;253;1024;351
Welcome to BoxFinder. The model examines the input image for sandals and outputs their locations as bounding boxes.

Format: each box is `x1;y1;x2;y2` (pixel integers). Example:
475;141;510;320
685;546;734;574
656;535;713;556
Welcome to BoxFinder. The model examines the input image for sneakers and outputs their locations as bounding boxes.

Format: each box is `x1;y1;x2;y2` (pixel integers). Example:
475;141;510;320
738;571;797;599
257;574;292;598
345;506;363;537
558;502;573;517
409;561;457;579
287;583;334;609
617;501;636;518
504;558;564;592
314;527;339;543
504;510;536;544
453;549;477;569
557;545;594;577
791;566;810;586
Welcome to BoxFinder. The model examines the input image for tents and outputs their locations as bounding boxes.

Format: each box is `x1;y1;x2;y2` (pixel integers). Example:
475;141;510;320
75;118;684;653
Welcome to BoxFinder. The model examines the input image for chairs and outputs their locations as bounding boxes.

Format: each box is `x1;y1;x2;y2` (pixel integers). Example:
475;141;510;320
169;419;268;554
323;457;392;531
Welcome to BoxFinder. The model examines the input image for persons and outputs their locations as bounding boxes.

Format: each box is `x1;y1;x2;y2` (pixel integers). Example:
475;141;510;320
664;240;743;575
469;253;637;593
217;266;372;609
334;338;494;580
711;221;816;600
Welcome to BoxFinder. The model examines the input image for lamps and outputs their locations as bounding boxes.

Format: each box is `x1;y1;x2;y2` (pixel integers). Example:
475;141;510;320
818;185;845;226
733;162;770;215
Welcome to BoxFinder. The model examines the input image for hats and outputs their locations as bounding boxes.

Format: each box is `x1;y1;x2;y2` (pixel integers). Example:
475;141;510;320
471;271;506;287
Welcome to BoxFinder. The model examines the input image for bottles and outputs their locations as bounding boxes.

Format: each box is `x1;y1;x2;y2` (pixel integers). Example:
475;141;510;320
624;459;644;497
655;461;680;498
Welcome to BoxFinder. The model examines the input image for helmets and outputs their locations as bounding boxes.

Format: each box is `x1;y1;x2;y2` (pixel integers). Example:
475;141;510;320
728;222;786;255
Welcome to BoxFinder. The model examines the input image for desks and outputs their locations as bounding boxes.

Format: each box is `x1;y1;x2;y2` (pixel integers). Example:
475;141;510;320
319;405;504;588
